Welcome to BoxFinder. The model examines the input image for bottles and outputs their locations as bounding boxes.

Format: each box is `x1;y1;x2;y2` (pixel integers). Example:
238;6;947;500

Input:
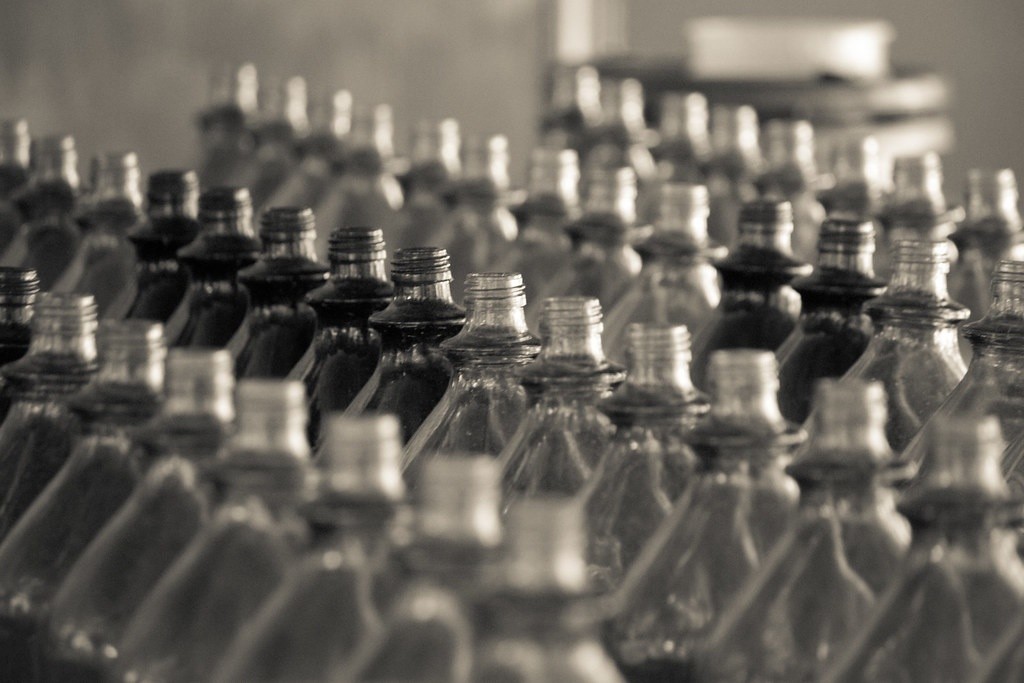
2;60;1023;683
